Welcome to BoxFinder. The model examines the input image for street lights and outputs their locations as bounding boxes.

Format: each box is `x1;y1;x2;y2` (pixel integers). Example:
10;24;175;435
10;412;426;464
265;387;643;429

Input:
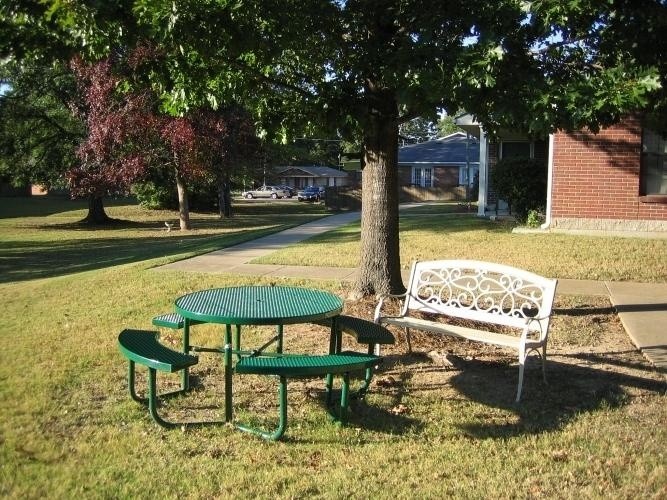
337;153;340;171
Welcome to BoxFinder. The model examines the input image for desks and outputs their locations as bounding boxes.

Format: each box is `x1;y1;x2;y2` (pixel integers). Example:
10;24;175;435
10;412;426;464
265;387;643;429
127;286;377;441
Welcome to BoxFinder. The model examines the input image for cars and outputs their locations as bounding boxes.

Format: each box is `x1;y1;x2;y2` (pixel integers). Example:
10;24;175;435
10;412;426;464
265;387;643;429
242;184;325;202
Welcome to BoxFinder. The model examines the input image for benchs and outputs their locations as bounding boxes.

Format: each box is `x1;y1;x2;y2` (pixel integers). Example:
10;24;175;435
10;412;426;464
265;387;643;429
152;313;395;345
374;259;559;402
117;329;197;373
236;350;384;375
